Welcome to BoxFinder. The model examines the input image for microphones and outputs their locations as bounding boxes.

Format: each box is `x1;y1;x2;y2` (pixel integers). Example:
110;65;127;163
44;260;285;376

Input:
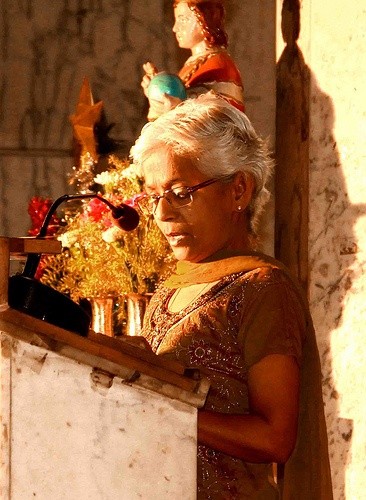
8;191;140;337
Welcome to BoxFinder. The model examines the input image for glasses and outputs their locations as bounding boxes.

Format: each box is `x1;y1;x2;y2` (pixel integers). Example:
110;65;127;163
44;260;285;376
134;175;222;217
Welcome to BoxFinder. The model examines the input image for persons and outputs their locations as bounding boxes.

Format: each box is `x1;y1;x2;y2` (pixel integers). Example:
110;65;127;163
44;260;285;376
138;1;247;122
84;89;304;499
146;72;187;122
